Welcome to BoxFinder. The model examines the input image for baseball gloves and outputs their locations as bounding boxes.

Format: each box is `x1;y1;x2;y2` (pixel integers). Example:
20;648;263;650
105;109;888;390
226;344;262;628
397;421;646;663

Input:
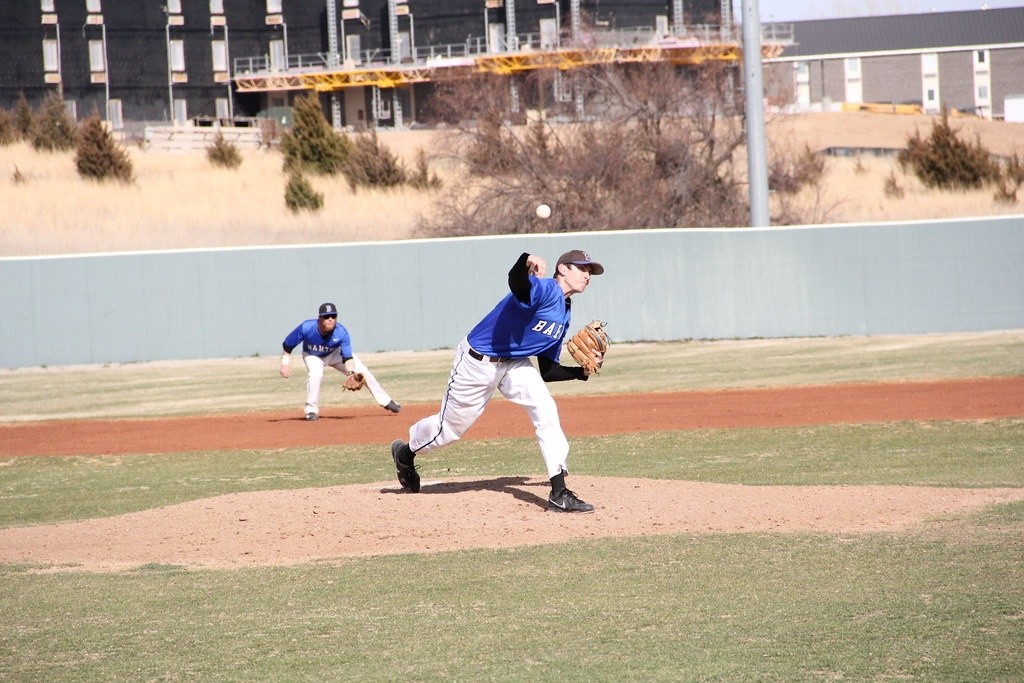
342;372;365;392
567;319;610;375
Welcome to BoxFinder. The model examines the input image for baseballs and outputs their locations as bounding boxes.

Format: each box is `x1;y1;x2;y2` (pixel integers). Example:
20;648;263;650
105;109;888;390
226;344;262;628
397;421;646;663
535;204;552;219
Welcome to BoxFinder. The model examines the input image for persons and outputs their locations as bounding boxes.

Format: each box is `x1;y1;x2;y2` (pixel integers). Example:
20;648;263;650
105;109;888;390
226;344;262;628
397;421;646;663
391;251;604;513
279;303;401;421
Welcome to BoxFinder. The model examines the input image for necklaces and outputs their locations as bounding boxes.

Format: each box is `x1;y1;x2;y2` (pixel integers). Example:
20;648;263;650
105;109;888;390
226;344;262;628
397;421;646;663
565;298;573;305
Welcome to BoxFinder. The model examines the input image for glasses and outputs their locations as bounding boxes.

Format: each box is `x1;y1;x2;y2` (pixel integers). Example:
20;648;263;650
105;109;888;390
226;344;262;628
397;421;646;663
319;314;337;319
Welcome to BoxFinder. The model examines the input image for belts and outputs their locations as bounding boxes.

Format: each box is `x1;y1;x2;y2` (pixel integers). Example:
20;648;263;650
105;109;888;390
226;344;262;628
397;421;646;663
469;348;511;363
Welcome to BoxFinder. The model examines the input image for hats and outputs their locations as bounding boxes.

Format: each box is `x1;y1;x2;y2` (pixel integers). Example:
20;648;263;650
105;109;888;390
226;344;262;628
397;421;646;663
556;250;604;276
319;303;337;315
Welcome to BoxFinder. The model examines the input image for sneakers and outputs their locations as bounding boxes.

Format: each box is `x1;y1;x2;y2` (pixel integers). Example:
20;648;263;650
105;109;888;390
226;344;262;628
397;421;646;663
391;439;421;493
547;488;595;513
306;412;318;421
384;400;401;413
